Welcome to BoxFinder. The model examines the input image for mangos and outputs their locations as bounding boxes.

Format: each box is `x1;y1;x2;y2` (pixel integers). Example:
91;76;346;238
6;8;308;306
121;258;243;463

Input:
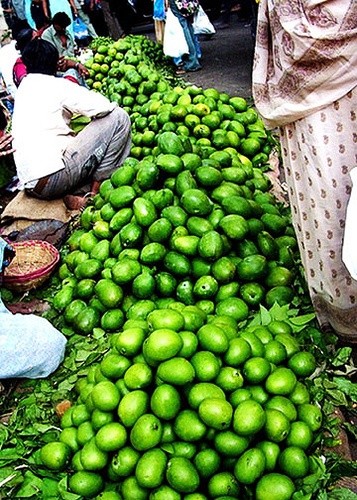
65;34;275;158
41;305;322;500
51;131;297;331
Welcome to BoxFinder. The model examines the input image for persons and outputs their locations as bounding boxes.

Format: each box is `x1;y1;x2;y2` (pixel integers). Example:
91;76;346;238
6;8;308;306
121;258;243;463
11;39;131;209
0;297;67;380
252;0;357;340
0;0;258;159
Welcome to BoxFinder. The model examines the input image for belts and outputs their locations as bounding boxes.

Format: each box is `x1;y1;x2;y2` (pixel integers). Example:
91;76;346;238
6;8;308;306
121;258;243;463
24;175;50;197
